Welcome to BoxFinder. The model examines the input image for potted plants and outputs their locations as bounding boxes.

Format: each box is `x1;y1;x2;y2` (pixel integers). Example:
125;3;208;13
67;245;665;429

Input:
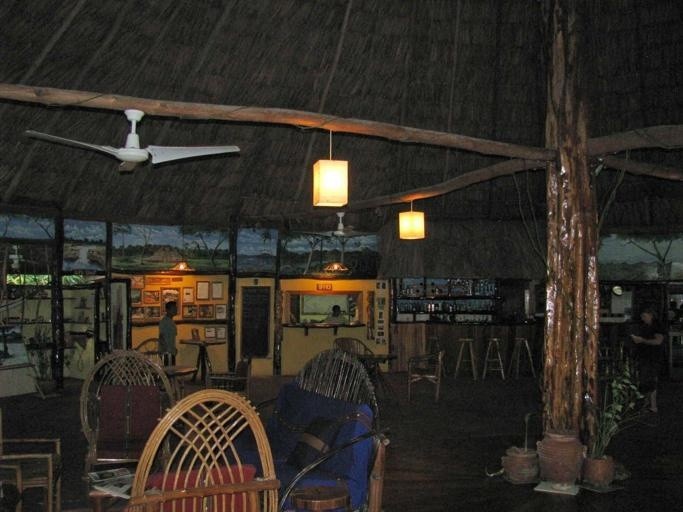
584;369;646;486
501;411;582;485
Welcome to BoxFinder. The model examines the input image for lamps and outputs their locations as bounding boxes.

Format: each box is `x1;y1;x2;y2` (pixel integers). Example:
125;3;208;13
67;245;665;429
398;201;425;240
312;129;348;208
320;239;348;274
169;228;194;273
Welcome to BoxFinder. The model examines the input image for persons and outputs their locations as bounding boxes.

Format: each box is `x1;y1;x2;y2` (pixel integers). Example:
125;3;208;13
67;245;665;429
627;308;664;415
325;305;350;325
159;300;180;366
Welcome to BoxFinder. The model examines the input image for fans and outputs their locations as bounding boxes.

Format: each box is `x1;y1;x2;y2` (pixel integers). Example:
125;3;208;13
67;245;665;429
22;110;240;175
288;212;378;242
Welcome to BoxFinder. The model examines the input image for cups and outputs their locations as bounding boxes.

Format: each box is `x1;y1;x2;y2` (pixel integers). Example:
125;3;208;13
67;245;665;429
191;328;199;342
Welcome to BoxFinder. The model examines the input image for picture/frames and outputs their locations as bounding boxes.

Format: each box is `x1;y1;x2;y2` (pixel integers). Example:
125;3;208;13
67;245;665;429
129;274;228;323
202;325;227;340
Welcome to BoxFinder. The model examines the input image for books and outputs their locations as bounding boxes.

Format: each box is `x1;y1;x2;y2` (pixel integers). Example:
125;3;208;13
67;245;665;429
88;467;132;483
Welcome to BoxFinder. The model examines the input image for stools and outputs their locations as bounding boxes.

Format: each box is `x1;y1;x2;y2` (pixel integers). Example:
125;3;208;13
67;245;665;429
453;337;537;382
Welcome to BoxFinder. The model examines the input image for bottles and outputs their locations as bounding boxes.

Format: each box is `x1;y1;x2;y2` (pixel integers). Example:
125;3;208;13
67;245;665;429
397;277;496;314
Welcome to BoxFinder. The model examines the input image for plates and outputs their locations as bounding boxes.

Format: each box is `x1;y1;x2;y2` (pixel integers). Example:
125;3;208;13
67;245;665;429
313;323;342;327
